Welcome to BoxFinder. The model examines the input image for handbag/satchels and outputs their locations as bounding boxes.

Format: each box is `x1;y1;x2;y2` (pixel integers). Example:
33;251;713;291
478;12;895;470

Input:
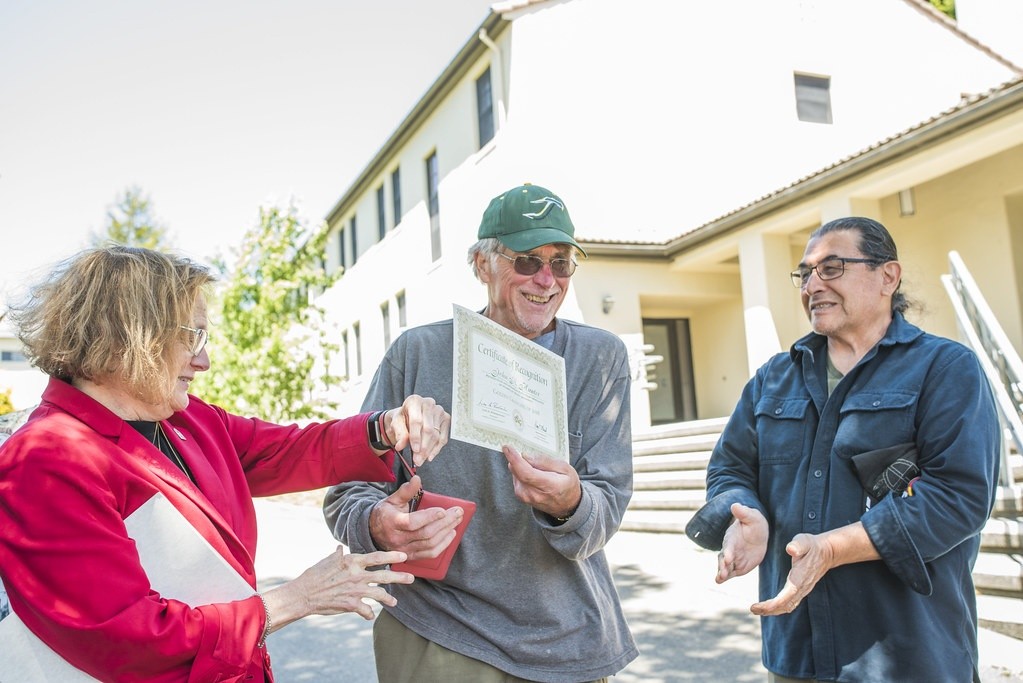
380;409;476;580
849;441;918;516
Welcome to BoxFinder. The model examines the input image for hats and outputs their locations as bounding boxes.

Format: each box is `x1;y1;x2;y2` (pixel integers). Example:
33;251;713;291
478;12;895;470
480;182;588;260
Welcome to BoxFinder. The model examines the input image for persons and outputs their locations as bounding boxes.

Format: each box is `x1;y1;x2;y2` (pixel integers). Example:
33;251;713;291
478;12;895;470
323;183;642;683
0;243;451;683
683;215;1002;683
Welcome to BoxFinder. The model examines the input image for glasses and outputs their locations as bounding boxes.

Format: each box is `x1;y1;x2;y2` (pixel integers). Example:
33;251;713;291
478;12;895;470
789;258;879;289
495;248;578;278
176;325;208;356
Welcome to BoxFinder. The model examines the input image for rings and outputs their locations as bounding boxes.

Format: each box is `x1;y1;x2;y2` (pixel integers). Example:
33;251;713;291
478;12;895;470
434;426;441;433
732;561;736;571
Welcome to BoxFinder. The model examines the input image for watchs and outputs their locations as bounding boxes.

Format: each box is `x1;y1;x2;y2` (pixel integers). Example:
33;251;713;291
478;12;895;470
368;411;395;450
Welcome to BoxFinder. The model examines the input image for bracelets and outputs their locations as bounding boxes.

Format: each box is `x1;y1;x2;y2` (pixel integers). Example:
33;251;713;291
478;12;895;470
544;511;576;527
253;592;271;648
